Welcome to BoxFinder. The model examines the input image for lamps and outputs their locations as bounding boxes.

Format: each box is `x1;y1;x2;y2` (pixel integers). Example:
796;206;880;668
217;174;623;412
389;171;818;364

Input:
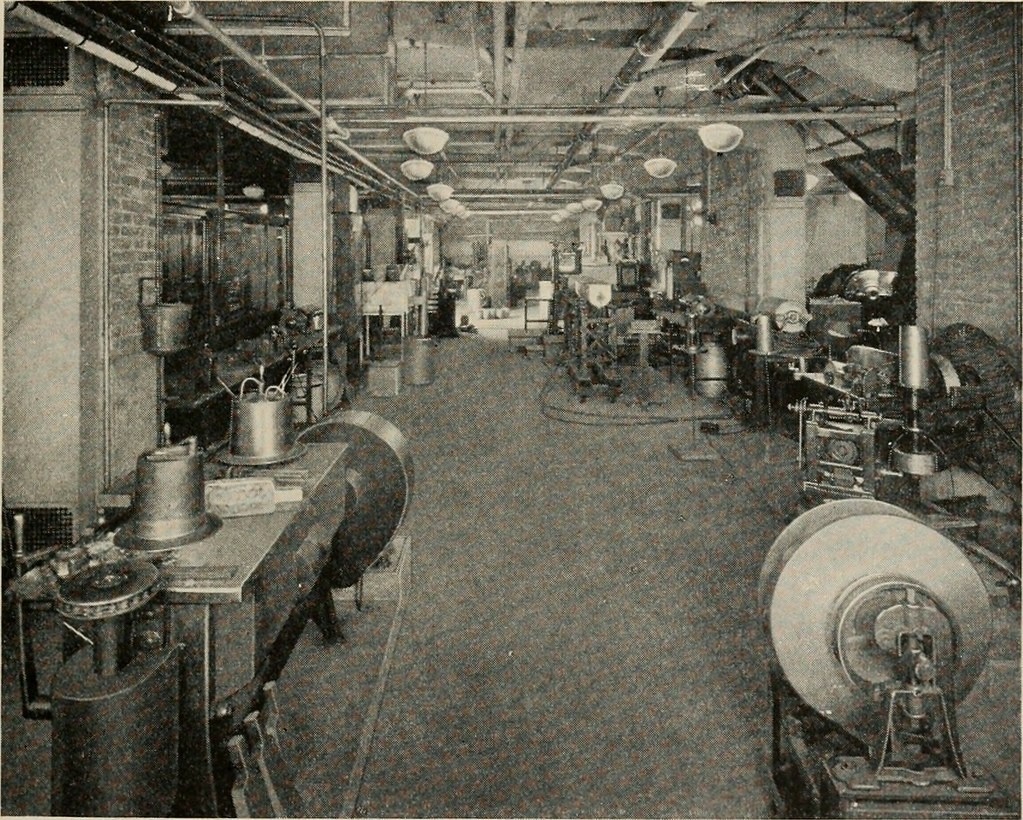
550;149;624;223
639;86;683;181
242;184;268;201
400;42;471;221
696;51;745;155
